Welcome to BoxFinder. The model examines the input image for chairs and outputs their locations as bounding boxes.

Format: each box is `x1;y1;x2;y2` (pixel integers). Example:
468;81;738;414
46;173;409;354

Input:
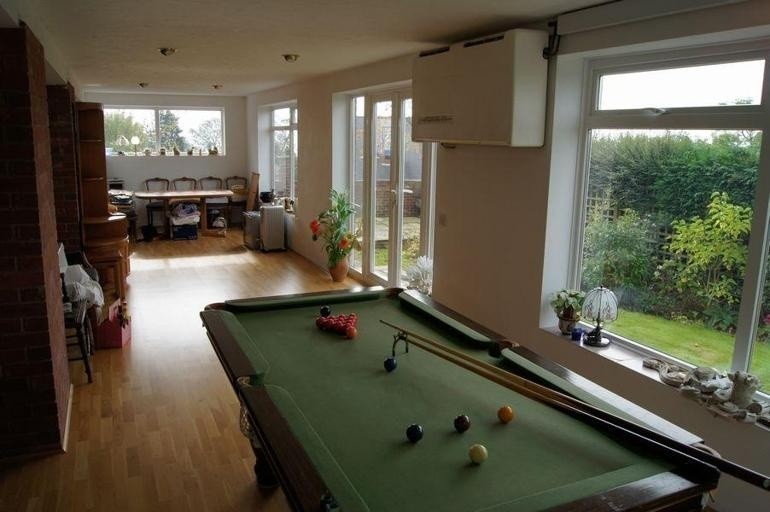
226;177;248;230
199;176;222;234
53;250;104;383
173;177;197;234
145;177;170;229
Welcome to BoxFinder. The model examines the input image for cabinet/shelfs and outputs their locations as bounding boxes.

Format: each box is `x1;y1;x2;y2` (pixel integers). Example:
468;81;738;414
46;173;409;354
74;101;110;218
83;212;130;282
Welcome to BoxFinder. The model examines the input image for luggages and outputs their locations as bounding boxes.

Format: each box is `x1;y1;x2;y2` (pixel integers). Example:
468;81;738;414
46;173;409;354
260;206;286;252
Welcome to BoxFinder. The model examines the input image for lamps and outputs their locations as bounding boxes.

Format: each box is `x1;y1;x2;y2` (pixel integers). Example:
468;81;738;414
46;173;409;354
113;136;129;155
131;135;139;155
581;286;619;347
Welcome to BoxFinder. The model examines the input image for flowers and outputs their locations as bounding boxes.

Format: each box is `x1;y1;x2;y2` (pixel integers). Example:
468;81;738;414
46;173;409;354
310;186;376;268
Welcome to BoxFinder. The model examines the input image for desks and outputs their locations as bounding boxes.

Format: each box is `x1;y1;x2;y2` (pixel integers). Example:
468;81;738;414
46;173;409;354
136;190;234;238
200;285;720;511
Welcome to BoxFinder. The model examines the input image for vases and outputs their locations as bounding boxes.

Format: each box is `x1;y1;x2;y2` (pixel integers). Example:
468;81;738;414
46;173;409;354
328;254;349;281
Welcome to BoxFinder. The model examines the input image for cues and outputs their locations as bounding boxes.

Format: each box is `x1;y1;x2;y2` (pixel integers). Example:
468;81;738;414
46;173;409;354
399;335;720;489
380;319;770;491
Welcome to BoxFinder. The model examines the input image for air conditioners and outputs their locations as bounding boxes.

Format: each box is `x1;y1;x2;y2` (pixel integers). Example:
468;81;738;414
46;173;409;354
408;27;554;148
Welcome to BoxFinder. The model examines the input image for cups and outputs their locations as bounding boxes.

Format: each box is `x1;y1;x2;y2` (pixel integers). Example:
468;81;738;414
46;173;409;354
572;327;583;340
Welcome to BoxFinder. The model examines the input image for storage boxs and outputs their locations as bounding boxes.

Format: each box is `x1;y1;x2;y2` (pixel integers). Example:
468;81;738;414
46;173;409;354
96;314;131;349
100;296;121;322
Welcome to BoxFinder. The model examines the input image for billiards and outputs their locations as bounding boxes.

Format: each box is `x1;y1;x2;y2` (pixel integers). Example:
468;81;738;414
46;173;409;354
497;406;512;423
384;358;396;371
316;312;357;338
320;306;330;316
406;424;423;441
469;445;487;464
455;414;470;433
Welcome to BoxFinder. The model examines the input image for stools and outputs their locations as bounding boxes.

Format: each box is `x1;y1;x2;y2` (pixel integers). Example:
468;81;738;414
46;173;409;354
85;248;129;304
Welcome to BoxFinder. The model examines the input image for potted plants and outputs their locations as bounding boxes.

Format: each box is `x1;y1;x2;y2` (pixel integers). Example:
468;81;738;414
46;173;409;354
549;287;586;334
